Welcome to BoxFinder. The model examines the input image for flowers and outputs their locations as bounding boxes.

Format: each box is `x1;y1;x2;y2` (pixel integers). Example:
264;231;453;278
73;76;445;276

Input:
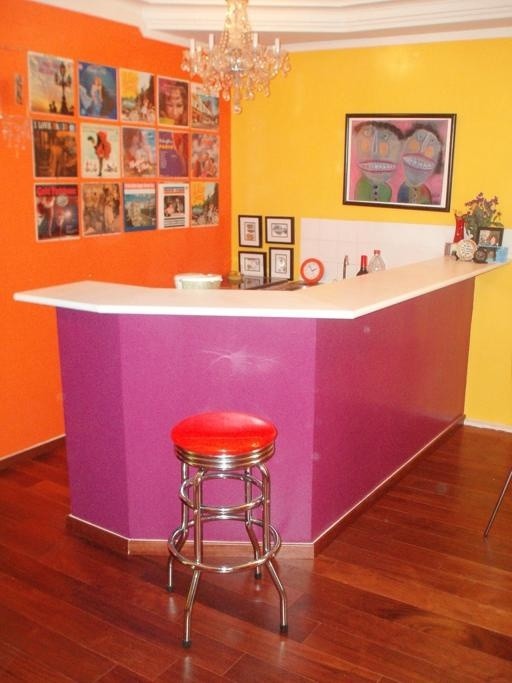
462;192;504;240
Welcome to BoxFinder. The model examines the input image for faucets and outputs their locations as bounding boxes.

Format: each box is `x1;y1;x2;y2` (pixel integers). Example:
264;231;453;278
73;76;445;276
343;255;349;278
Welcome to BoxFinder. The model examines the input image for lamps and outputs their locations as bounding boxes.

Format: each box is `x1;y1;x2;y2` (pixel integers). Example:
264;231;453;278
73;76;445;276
180;0;291;115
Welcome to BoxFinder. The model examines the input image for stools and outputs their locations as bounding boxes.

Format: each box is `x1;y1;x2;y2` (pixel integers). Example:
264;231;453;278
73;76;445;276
167;411;288;648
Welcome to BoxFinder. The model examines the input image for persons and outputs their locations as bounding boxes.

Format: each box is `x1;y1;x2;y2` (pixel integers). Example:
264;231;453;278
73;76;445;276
160;81;188;125
90;74;105;118
165;202;175;215
122;127;156;175
141;94;149;122
100;186;116;232
192;152;216;176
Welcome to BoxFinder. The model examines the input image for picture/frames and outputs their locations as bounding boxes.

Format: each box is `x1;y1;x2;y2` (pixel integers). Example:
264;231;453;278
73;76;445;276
239;275;266;290
269;277;289;286
238;215;262;248
343;113;457;212
238;251;266;282
476;227;504;246
268;247;294;281
482;247;497;261
265;216;294;244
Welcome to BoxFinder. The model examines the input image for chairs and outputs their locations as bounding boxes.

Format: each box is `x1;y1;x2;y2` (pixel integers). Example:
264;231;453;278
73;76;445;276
483;470;512;536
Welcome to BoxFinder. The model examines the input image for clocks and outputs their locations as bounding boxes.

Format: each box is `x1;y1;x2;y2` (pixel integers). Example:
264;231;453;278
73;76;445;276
300;258;324;286
472;247;488;264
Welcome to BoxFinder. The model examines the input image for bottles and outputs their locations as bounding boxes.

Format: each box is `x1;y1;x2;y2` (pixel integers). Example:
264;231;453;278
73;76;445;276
356;255;369;276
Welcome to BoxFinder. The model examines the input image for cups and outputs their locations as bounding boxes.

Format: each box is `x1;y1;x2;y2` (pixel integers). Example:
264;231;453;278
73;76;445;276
495;246;508;263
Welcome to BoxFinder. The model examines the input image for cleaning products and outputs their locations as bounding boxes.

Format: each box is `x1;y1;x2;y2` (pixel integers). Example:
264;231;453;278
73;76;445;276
356;254;367;274
